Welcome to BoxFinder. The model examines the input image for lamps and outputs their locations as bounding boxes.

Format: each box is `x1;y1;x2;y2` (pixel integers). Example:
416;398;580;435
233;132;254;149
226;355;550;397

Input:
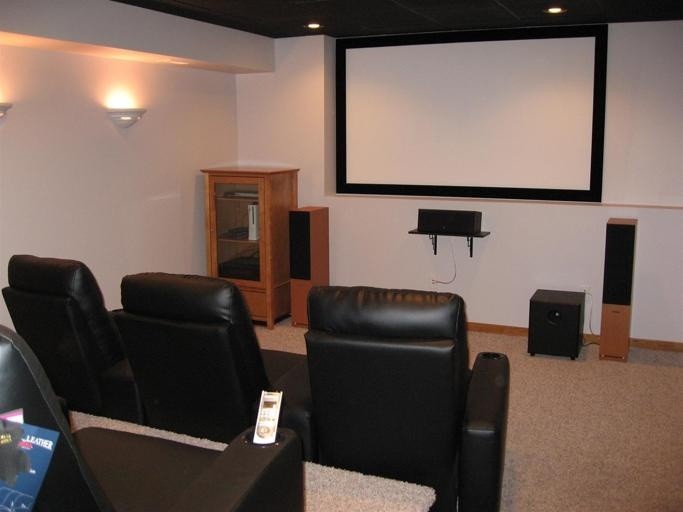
105;103;146;130
0;98;14;119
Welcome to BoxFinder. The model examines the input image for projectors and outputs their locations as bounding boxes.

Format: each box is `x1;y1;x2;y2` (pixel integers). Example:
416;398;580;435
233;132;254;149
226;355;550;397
417;208;482;235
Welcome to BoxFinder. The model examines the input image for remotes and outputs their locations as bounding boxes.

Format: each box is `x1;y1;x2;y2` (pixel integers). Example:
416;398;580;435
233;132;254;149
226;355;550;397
252;390;283;446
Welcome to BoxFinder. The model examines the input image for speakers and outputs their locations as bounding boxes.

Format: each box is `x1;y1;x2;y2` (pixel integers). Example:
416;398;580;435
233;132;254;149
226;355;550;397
599;218;639;363
287;207;329;326
527;288;585;359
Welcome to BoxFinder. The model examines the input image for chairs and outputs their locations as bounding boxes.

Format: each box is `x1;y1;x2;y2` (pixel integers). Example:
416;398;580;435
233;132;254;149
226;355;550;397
304;284;509;512
1;252;144;424
114;270;315;459
2;326;306;511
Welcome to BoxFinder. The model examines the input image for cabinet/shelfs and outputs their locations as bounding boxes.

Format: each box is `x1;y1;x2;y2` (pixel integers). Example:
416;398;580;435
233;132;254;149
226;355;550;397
201;158;299;329
